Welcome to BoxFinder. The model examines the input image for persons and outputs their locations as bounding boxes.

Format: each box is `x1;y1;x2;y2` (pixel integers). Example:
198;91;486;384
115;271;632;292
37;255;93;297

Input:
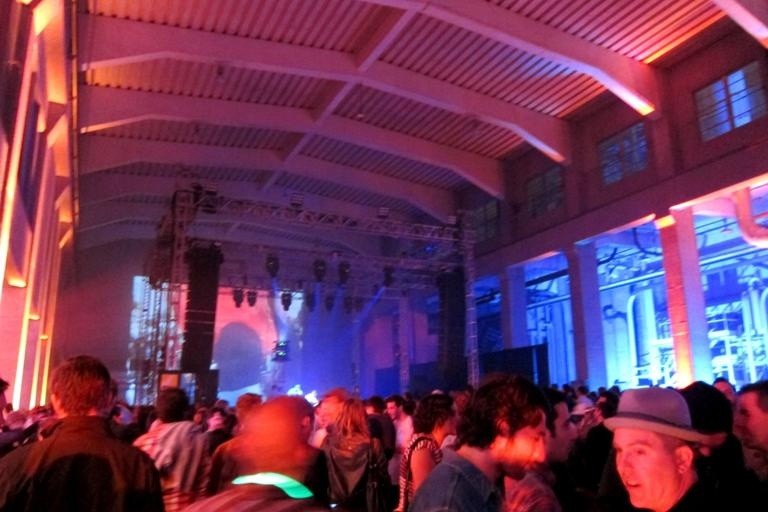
0;353;767;512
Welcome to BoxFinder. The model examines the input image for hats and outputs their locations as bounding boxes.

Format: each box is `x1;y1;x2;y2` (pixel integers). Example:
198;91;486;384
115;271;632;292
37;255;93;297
603;389;727;446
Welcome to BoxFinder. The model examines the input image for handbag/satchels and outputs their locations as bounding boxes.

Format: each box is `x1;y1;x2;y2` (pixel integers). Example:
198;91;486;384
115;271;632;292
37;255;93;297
367;466;391;511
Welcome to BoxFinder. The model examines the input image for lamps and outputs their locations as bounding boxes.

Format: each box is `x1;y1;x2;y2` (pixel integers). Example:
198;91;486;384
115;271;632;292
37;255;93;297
232;254;399;314
287;191;306;208
200;182;219;214
443;214;458;225
379;203;393;219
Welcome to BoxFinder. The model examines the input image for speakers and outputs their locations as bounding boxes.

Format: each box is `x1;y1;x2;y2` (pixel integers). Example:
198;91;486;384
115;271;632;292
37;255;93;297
180;256;220;371
195;370;219;405
435;275;466;372
